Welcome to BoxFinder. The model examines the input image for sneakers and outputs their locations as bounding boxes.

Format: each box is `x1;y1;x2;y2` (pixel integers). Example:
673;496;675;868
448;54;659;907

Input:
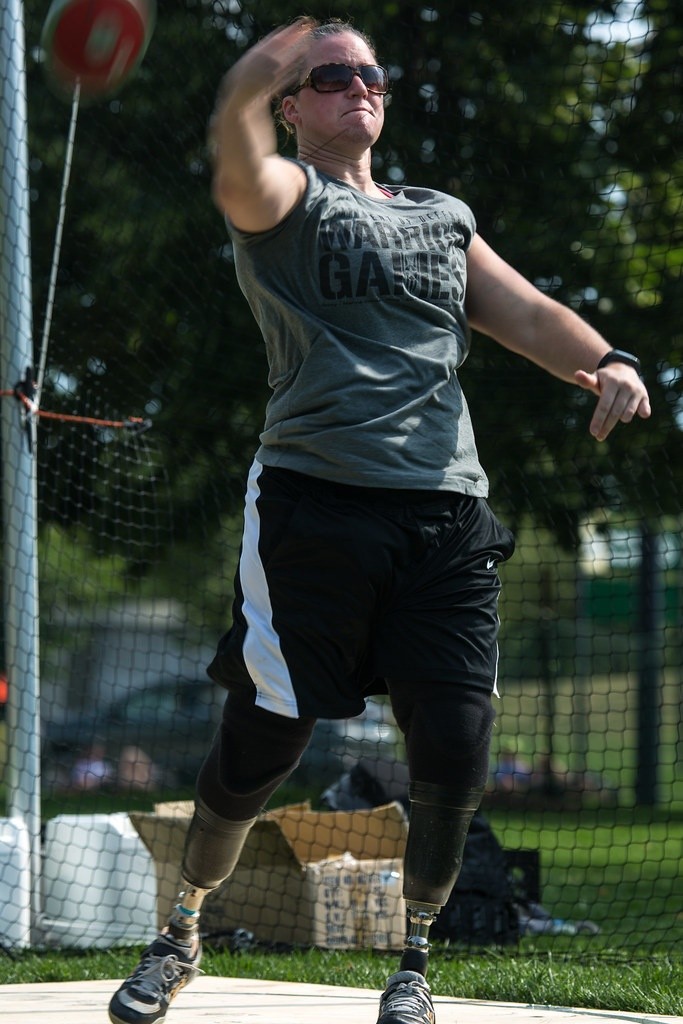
109;923;206;1024
376;971;435;1024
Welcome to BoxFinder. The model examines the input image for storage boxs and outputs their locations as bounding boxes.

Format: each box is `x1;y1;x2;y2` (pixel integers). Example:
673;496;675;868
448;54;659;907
0;813;158;951
129;799;411;949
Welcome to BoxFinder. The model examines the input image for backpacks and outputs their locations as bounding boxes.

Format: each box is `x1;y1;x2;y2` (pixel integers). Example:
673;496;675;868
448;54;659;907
407;818;528;946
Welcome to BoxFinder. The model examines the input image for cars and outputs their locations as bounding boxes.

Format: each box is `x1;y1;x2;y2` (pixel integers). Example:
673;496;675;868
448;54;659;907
40;676;409;776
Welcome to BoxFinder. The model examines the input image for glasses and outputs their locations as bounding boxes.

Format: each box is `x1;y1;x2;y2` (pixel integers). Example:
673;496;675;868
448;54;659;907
291;62;389;94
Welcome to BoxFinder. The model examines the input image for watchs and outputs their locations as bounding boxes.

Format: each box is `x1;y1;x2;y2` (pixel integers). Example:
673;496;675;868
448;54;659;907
596;349;642;378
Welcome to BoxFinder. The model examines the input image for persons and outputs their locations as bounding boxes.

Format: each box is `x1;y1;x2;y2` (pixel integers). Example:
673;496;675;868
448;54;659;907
104;19;651;1024
44;683;606;808
